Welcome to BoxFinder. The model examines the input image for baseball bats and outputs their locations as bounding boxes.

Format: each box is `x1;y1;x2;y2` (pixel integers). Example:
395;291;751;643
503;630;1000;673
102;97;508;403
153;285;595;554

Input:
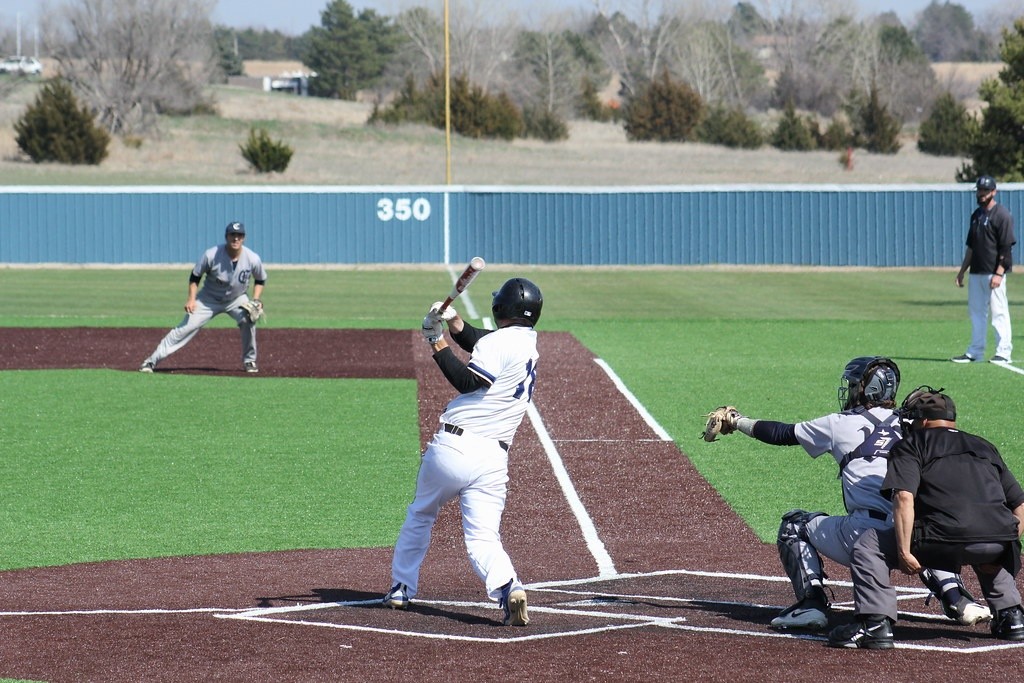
437;257;486;315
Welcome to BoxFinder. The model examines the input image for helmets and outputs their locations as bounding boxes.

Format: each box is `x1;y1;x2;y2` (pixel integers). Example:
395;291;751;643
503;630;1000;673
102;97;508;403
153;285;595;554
977;175;996;190
492;277;543;327
838;356;900;410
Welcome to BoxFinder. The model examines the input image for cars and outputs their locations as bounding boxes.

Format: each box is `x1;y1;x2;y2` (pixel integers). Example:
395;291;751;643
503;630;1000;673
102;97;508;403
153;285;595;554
0;55;44;75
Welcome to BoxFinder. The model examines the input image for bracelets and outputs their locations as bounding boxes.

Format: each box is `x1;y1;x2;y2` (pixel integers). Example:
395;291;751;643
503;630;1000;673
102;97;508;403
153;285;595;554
995;272;1002;277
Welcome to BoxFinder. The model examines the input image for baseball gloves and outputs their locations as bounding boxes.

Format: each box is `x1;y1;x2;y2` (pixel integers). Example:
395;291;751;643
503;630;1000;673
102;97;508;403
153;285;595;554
238;299;267;324
697;404;750;443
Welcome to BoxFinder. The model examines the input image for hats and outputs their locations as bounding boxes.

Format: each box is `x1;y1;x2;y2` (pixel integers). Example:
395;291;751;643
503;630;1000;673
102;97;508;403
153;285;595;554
225;221;246;234
914;392;956;420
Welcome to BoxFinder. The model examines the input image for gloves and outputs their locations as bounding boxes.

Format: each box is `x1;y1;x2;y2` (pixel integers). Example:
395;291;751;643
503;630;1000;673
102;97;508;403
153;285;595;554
431;302;456;321
422;310;443;343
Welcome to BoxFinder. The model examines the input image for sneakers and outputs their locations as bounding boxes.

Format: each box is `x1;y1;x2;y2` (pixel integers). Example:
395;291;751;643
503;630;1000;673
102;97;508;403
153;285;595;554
499;579;531;625
949;353;975;362
949;596;993;625
244;361;259;372
989;355;1012;362
139;362;152;372
989;608;1024;640
771;603;829;631
383;583;409;610
835;620;894;648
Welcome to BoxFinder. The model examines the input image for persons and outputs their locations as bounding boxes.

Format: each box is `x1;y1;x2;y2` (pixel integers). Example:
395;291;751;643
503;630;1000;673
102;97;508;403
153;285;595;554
829;386;1024;649
138;222;267;372
951;175;1017;365
382;279;543;625
703;356;994;628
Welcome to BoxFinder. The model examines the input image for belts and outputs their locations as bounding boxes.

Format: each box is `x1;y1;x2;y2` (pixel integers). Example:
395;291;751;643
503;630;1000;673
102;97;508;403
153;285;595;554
445;422;508;452
